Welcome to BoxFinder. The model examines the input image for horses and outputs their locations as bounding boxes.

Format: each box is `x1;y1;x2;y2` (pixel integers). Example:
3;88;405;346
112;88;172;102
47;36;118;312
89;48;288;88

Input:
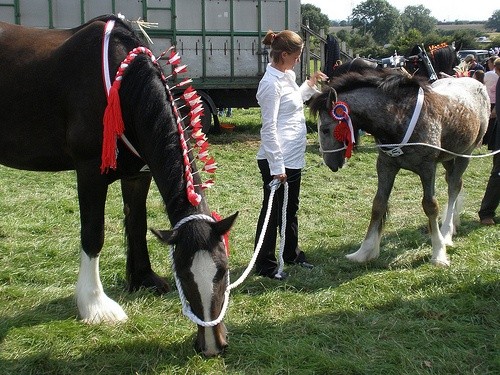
304;42;492;267
0;15;239;360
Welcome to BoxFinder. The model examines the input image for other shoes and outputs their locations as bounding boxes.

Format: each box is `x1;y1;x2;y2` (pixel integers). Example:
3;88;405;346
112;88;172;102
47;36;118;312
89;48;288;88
273;273;283;280
301;261;314;269
481;218;495;226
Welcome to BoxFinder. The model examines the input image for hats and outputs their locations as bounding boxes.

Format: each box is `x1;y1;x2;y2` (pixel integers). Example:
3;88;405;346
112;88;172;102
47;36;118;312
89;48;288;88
463;54;476;63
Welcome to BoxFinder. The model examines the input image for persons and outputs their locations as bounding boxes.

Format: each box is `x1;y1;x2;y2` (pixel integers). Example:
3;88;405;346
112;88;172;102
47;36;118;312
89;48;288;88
465;46;500;225
255;30;331;279
